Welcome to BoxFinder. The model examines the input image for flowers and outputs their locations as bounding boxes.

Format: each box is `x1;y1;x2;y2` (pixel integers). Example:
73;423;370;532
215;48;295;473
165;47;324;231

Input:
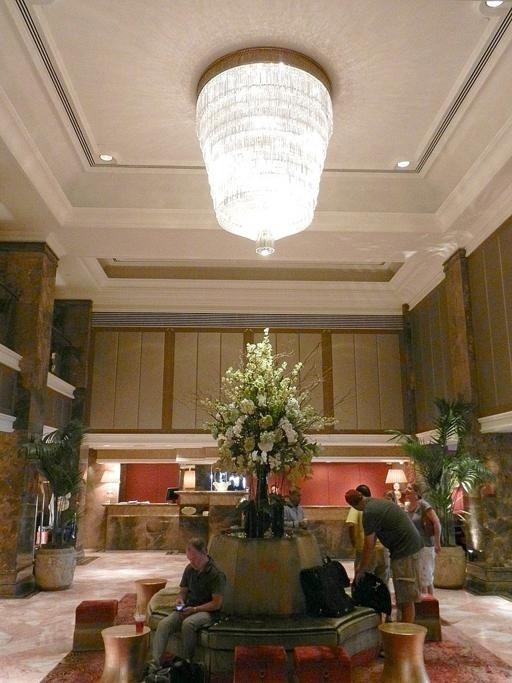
182;324;356;496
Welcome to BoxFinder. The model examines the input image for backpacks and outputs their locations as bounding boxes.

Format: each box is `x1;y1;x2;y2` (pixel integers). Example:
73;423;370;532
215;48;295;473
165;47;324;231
299;556;357;618
351;570;393;616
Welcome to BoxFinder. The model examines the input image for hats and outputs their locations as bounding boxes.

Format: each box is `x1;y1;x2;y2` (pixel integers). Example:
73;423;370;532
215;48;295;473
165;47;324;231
345;488;362;505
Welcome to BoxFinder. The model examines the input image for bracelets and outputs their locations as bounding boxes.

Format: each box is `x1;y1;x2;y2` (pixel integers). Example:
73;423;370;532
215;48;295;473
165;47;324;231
192;606;198;613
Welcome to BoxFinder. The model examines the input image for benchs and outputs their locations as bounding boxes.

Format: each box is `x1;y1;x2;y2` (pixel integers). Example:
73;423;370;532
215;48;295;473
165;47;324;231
146;580;384;679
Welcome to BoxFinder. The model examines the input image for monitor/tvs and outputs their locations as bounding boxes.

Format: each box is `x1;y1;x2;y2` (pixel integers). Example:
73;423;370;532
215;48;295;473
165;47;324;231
166;487;179;503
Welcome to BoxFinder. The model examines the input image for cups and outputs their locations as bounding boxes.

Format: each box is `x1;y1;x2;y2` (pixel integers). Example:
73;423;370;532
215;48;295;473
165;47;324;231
133;614;146;635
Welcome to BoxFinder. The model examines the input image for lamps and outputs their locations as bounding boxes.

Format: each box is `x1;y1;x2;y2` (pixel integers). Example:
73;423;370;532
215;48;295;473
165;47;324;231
385;468;408;506
100;470;120;503
194;47;336;257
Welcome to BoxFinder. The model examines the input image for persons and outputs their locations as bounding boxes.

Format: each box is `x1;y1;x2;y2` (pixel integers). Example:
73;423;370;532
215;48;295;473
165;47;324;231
342;489;425;658
345;483;390;625
226;479;239;490
383;489;400;506
404;482;442;595
282;487;311;529
142;535;227;673
268;483;283;497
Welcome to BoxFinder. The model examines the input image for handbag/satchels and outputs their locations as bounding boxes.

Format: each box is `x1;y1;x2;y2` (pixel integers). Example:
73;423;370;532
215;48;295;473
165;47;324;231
322;554;350;587
142;656;202;683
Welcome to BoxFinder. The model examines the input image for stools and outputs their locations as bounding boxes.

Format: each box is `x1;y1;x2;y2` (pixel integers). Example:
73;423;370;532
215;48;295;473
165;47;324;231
73;599;119;652
378;622;428;682
414;592;441;642
133;578;168;622
233;641;352;682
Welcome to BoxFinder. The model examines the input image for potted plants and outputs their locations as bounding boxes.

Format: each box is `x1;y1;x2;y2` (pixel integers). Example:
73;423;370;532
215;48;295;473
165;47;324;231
385;392;496;589
19;416;94;591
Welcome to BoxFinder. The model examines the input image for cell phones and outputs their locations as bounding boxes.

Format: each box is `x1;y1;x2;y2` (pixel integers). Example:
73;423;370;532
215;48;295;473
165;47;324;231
175;604;185;612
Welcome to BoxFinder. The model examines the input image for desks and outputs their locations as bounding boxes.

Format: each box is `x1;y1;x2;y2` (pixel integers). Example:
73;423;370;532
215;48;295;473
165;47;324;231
98;622;151;682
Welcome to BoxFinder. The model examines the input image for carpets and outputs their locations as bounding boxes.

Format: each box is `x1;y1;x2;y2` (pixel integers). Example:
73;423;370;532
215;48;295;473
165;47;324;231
39;592;511;682
